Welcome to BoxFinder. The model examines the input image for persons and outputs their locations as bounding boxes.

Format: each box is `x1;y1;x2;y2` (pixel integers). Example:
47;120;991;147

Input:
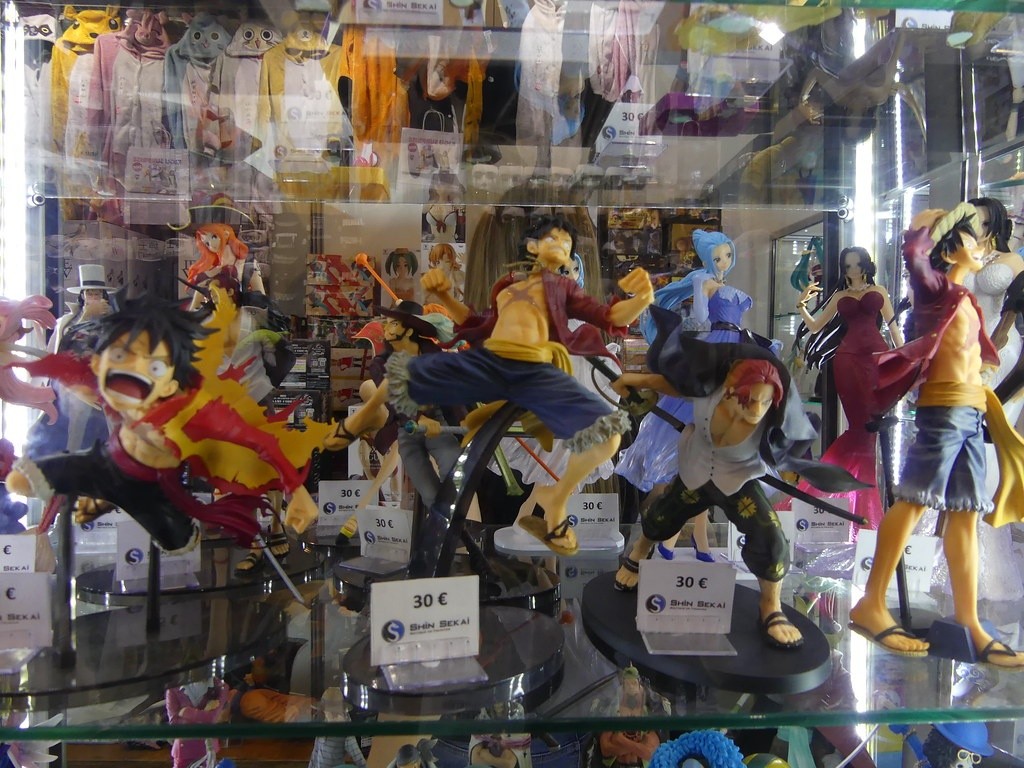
0;196;1024;768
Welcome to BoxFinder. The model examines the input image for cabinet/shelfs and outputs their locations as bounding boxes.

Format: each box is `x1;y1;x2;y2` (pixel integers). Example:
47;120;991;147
0;0;1024;768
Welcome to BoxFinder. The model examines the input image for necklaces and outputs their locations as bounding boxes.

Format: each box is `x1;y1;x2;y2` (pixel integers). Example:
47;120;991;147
711;277;726;283
979;250;1001;265
847;284;870;293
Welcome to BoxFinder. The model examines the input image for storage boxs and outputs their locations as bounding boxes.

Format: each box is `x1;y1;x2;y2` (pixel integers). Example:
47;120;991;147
277;339;332;389
625;321;644;339
663;197;722;225
663;223;724;254
273;390;332;427
331;348;377;380
420;242;467;306
420;171;467;243
615;334;650;373
305;285;375;318
331;379;365;412
601;227;663;254
608;254;669;278
306;316;373;349
381;246;422;315
304;253;374;285
607;202;662;229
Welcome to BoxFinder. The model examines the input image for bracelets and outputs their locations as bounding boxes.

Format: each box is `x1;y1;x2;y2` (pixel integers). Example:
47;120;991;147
795;302;807;309
